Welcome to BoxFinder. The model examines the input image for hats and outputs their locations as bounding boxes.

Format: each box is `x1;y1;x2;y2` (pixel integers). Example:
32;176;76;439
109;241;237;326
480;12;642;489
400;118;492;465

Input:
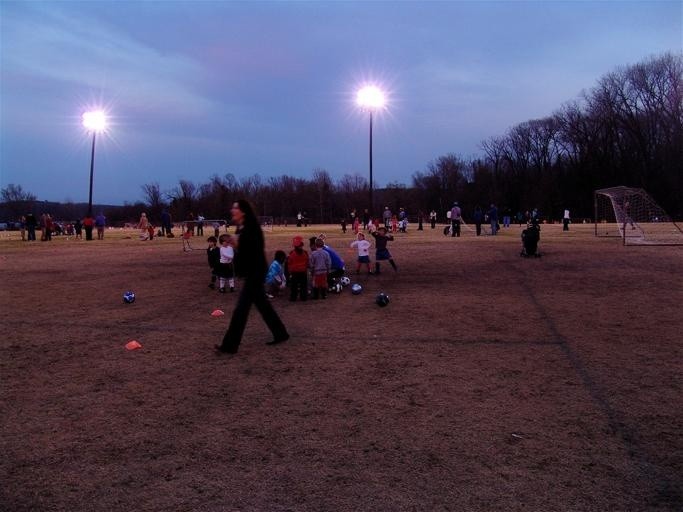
292;236;302;248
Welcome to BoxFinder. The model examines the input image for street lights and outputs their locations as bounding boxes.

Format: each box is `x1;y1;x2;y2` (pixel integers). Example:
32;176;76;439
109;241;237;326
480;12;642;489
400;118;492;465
81;110;107;217
354;82;387;218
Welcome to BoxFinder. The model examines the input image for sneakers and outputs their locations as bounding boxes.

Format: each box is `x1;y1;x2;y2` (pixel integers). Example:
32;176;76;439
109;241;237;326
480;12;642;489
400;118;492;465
208;282;236;292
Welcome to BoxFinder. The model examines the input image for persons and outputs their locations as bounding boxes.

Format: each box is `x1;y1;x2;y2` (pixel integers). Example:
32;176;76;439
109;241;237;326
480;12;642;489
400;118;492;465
351;227;398;276
21;209;105;241
215;200;290;355
563;209;570;231
416;209;424;230
297;211;308;227
341;206;408;232
138;209;205;241
207;234;235;292
430;210;437;229
447;201;537;237
620;194;636;230
266;233;346;302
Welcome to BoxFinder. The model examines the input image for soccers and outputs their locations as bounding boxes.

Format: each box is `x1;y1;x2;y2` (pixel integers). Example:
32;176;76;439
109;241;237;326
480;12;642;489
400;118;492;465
340;276;350;285
377;293;389;307
332;283;342;293
123;291;135;302
352;284;361;294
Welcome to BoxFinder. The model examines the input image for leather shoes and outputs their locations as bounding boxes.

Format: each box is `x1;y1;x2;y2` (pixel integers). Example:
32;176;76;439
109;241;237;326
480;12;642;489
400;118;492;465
214;344;222;350
266;338;279;345
